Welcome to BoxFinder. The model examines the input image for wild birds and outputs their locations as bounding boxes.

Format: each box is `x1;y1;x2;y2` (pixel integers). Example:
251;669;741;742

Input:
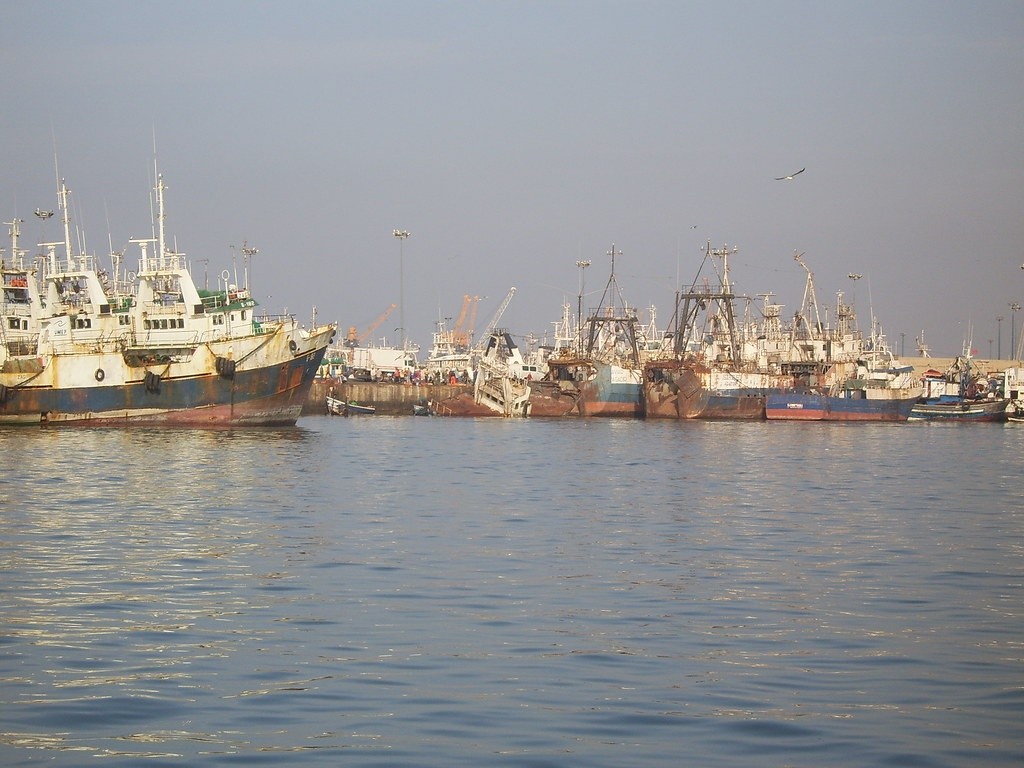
775;168;805;180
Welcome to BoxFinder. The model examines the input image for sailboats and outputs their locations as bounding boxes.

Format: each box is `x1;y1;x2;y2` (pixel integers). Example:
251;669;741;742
0;141;1024;426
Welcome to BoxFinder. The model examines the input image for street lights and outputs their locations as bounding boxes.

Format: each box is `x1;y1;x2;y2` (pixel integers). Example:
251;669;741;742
239;240;259;296
995;314;1003;360
848;272;863;308
1007;301;1022;360
899;332;906;357
988;339;994;359
392;228;411;346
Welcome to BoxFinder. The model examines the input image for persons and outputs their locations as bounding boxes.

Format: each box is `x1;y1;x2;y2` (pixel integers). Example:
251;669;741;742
369;366;468;386
527;372;532;381
